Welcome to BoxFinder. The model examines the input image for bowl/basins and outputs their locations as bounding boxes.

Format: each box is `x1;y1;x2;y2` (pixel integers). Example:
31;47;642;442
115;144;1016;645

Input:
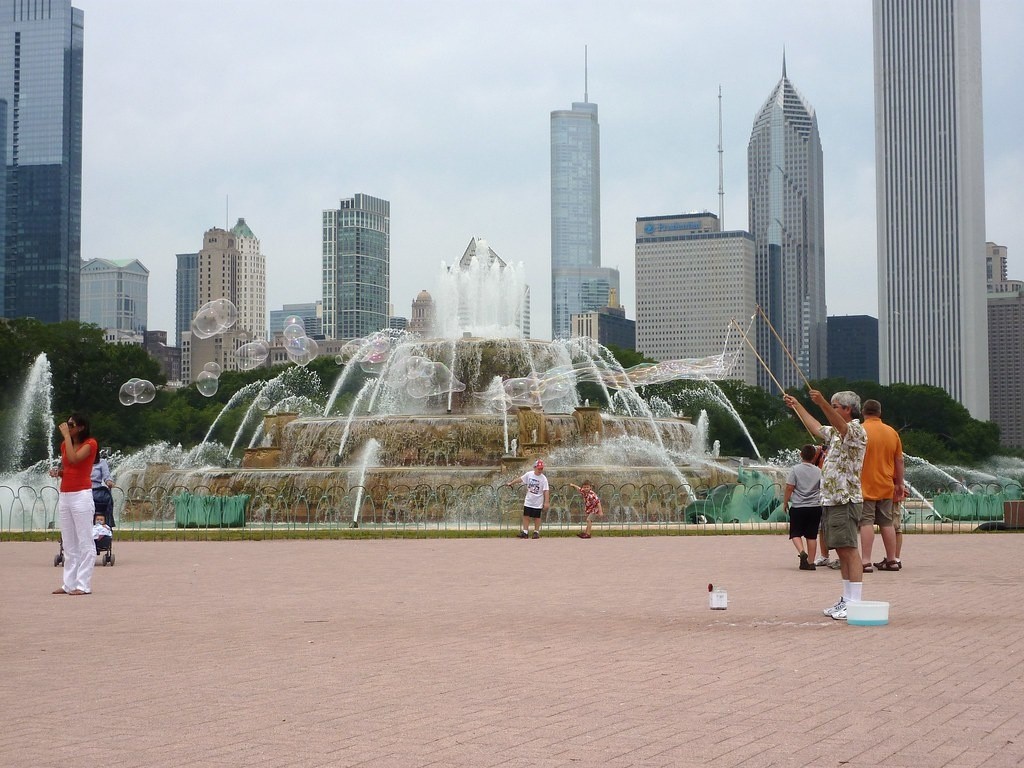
845;600;890;625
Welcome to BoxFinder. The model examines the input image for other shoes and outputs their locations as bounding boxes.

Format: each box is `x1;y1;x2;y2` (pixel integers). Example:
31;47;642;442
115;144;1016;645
806;563;816;570
532;532;539;539
52;588;66;595
69;590;91;595
576;533;591;538
797;551;808;569
517;531;529;538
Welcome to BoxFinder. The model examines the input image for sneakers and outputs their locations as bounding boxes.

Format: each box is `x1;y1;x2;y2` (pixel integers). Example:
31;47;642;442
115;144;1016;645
824;597;846;616
831;606;848;620
813;556;830;565
830;559;841;570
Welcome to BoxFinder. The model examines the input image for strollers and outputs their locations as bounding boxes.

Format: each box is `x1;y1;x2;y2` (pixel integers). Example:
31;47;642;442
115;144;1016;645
54;480;116;566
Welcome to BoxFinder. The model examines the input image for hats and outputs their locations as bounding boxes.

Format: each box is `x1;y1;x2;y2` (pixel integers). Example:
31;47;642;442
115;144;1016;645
533;460;543;469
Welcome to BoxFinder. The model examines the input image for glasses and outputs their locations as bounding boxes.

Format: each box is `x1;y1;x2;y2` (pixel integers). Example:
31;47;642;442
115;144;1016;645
67;422;77;429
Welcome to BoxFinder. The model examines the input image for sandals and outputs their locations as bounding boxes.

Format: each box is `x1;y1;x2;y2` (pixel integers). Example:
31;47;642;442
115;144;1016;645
878;560;899;572
862;563;873;573
873;558;902;569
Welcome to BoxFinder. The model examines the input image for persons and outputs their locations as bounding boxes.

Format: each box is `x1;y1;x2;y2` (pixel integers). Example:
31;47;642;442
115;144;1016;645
507;460;549;539
784;445;822;570
95;515;110;542
571;480;603;538
49;413;98;595
800;438;841;569
860;400;910;572
784;390;868;620
91;450;114;488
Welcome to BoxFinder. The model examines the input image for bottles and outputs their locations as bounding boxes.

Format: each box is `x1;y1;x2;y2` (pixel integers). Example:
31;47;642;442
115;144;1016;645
94;521;101;539
51;466;58;486
